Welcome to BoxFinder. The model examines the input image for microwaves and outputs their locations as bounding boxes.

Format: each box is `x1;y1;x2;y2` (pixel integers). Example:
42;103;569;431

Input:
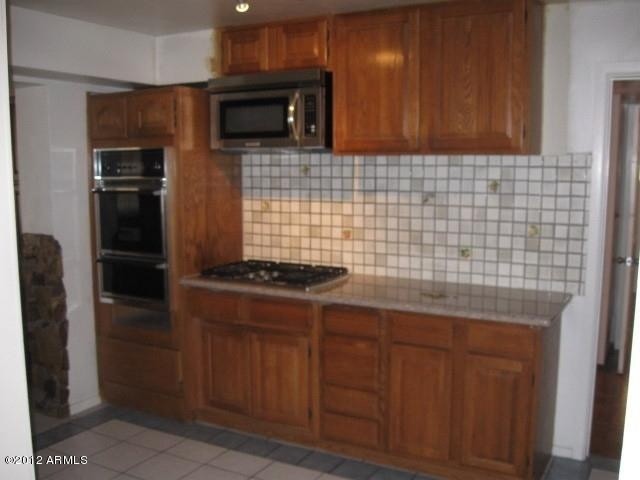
208;67;334;153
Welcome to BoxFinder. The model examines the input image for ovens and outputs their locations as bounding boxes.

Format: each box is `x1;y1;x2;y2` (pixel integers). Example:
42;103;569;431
92;145;171;309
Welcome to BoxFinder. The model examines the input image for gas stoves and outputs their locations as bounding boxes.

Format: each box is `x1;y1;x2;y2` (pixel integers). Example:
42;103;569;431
196;258;348;293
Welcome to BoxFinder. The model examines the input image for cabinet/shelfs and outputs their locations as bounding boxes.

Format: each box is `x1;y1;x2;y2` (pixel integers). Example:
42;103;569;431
186;289;319;453
86;87;242;414
331;5;420;153
315;302;382;467
387;311;561;479
419;2;544;155
222;14;332;76
